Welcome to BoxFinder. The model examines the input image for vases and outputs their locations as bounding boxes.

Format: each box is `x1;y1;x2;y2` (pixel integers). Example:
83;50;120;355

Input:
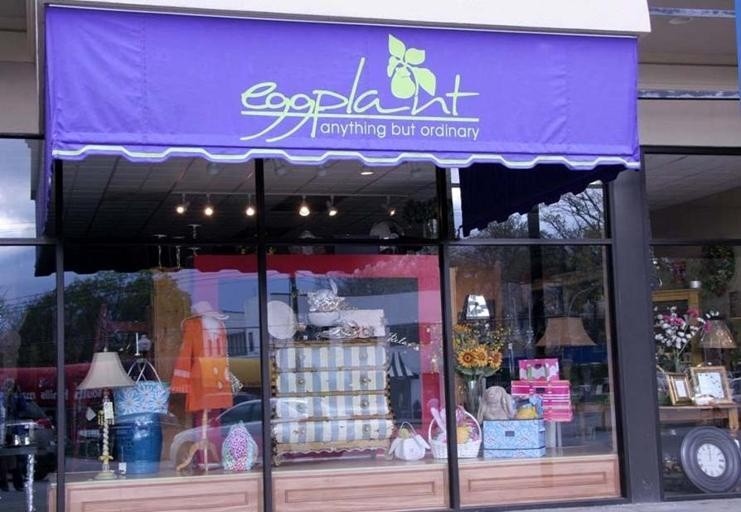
464;382;480;418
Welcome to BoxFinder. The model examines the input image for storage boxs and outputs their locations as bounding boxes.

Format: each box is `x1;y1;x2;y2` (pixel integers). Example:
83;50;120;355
482;358;575;458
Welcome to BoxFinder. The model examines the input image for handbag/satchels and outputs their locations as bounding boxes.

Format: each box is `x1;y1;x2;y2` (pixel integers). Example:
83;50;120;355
112;358;170;419
388;421;430;461
510;358;575;422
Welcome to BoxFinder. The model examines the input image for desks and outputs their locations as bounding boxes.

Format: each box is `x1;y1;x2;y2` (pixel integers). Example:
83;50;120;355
659;404;741;434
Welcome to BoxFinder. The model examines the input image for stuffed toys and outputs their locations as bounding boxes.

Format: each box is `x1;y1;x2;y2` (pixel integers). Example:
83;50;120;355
478;385;515;424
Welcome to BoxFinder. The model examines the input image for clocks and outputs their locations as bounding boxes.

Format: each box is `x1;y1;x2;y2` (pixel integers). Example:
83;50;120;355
680;426;741;493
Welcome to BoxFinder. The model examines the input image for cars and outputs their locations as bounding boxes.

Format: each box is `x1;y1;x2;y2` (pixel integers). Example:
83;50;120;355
0;394;58;481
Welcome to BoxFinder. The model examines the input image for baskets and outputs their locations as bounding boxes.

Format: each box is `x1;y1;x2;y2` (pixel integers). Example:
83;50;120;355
428;405;482;458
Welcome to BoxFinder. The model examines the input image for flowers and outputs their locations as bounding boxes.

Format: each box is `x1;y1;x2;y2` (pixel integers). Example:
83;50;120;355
654;305;720;371
452;310;514;380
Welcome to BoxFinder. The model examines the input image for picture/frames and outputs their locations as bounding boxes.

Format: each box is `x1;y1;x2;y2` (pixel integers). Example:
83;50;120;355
666;372;691;406
690;365;734;405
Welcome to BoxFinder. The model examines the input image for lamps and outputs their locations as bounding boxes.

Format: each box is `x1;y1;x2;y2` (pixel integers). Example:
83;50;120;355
75;351;139;481
170;191;409;218
695;319;738;365
534;317;599;347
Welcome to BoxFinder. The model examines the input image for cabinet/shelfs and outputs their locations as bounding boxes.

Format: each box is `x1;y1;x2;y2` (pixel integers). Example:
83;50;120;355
268;339;395;466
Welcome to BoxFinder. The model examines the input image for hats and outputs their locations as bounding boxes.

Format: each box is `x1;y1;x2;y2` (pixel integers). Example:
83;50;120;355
180;301;229;332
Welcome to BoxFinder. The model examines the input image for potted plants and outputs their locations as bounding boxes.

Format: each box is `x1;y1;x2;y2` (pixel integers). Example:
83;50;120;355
685;255;702;288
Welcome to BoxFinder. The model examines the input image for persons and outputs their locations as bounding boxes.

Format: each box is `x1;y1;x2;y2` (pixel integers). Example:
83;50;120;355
171;301;235;412
